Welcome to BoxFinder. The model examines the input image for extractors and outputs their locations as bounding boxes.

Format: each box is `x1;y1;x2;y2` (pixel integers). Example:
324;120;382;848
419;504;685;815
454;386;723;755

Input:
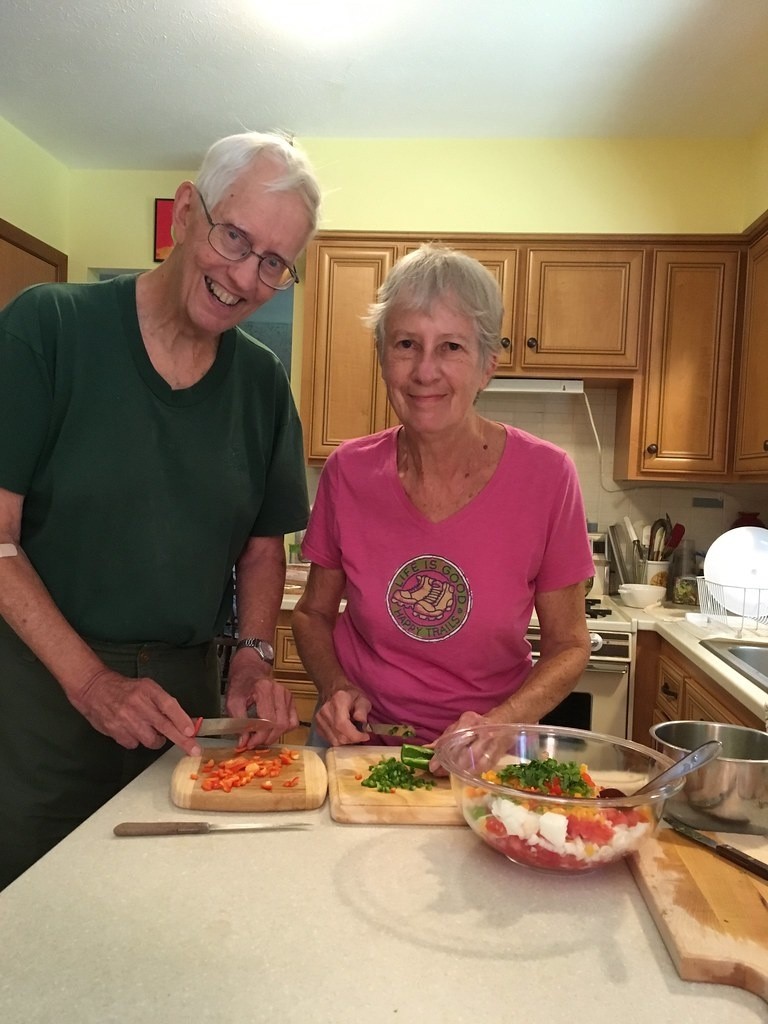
482;379;585;394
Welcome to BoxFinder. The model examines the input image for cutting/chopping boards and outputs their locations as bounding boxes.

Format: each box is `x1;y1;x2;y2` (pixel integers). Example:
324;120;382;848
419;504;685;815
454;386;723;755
325;745;532;826
170;748;327;813
625;805;767;1005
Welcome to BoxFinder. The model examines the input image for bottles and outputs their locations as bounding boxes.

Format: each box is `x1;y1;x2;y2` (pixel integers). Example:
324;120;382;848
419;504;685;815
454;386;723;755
730;512;765;530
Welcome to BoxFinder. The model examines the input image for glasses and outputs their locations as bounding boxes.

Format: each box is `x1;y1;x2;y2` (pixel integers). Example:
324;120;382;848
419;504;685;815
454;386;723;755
195;186;298;289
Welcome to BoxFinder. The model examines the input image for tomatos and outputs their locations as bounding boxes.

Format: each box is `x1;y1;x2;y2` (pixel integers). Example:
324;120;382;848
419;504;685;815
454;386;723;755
485;805;646;872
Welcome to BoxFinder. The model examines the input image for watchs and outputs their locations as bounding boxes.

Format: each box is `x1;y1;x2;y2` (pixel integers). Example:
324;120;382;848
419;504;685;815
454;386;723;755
236;638;275;665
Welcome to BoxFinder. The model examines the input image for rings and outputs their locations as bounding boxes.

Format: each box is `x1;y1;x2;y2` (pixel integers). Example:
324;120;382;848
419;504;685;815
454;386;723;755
484;752;496;764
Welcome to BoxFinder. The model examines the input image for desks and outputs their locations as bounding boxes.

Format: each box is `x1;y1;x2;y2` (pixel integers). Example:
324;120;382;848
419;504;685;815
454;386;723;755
1;739;768;1024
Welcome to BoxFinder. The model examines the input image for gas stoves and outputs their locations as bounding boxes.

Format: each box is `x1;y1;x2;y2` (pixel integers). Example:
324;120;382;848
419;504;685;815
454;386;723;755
524;533;637;662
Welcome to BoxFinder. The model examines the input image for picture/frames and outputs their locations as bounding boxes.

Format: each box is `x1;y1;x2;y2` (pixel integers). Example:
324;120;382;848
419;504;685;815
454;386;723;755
153;198;175;264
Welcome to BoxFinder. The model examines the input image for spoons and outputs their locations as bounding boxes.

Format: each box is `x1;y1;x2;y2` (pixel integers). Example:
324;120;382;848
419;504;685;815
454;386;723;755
595;740;724;800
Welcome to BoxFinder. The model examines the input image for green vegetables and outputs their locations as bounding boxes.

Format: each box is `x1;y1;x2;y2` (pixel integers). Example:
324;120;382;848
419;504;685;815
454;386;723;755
497;756;592;797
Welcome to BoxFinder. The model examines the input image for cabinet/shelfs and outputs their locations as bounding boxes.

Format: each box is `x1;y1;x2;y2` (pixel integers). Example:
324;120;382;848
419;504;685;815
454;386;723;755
632;243;744;477
651;634;748;752
731;230;768;481
298;232;402;464
404;246;644;374
271;608;320;744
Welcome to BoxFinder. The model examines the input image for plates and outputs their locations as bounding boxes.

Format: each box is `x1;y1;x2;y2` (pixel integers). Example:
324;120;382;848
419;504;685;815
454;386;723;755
703;526;768;616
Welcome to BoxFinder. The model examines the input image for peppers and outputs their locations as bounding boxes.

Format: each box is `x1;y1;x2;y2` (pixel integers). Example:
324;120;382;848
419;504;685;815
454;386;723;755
354;724;441;794
191;744;301;792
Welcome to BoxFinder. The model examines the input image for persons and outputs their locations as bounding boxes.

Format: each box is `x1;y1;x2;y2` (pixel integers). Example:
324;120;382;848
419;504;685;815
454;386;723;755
290;247;594;778
0;132;321;890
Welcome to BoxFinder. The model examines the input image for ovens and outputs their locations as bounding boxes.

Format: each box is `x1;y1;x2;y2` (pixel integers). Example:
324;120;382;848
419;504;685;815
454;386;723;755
532;656;636;742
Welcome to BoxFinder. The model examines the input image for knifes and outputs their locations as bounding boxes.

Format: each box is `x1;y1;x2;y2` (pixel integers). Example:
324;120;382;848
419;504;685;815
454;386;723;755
114;821;316;836
354;721;417;738
624;516;685;561
190;718;274;737
663;816;768;881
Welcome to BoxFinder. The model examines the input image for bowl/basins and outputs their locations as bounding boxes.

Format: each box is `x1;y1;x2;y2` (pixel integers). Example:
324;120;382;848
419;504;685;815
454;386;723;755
617;583;667;608
648;721;768;836
435;722;687;878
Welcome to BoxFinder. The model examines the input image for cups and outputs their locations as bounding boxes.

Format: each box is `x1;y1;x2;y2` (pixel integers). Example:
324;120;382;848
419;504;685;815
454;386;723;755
639;560;673;589
669;538;697;605
